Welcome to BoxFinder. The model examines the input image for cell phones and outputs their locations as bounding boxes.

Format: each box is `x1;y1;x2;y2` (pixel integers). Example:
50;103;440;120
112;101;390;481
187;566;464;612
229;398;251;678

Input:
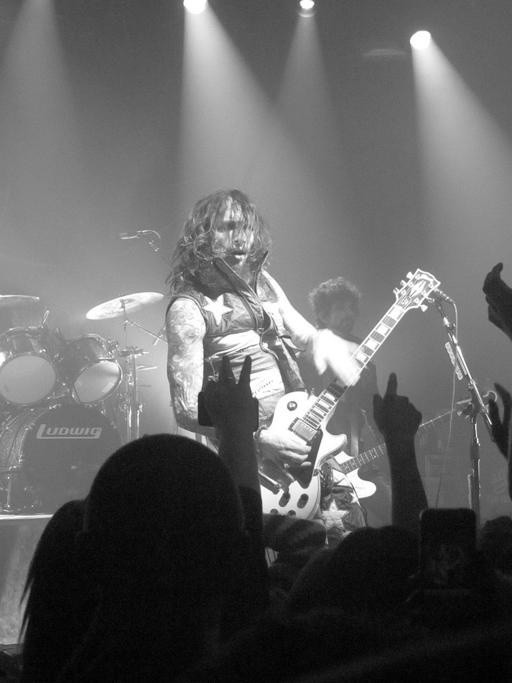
197;390;261;433
417;505;477;597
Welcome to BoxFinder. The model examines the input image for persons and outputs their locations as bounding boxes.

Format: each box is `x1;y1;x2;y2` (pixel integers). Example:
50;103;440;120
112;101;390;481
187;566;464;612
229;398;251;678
16;356;512;683
163;184;363;545
481;261;512;339
295;274;383;456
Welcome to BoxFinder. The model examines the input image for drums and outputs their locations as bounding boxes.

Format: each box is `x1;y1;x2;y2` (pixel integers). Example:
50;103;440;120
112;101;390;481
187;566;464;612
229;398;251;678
52;336;124;406
0;404;124;512
0;328;56;406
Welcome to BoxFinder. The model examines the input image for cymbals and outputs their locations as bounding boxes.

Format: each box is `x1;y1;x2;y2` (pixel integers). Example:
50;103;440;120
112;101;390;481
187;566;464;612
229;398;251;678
87;293;162;322
0;295;40;307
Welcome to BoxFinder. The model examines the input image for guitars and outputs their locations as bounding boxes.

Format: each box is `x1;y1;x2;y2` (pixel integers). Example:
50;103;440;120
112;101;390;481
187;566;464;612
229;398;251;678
258;267;442;519
335;389;497;501
